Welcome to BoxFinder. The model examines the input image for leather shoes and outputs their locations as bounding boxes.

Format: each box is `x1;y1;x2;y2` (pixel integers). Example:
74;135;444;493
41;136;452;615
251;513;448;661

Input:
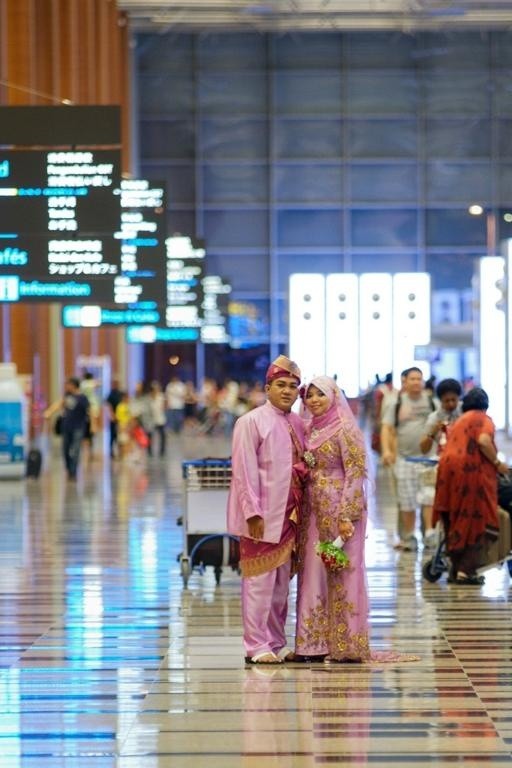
325;654;363;663
285;652;329;662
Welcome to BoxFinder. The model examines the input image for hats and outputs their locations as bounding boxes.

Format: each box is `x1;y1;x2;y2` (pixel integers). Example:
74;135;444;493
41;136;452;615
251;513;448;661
265;354;301;385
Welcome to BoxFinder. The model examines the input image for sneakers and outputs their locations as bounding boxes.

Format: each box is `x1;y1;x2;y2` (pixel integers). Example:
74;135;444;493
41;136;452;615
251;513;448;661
404;536;418;552
424;528;439;549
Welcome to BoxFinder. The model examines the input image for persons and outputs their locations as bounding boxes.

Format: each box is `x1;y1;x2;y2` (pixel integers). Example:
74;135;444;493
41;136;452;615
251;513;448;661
43;376;96;480
293;377;369;663
227;355;305;664
82;372;265;461
374;369;442;553
419;378;512;583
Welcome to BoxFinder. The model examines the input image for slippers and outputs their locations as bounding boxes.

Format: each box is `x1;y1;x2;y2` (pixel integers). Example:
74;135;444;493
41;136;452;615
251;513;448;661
244;648;292;664
447;569;484;584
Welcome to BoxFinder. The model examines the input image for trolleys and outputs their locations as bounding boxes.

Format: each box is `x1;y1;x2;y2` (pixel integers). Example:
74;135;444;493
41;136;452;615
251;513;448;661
405;457;511;582
180;458;241;590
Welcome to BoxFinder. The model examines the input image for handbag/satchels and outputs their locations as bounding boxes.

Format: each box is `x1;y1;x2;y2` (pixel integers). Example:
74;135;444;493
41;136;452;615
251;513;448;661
55;416;67;434
